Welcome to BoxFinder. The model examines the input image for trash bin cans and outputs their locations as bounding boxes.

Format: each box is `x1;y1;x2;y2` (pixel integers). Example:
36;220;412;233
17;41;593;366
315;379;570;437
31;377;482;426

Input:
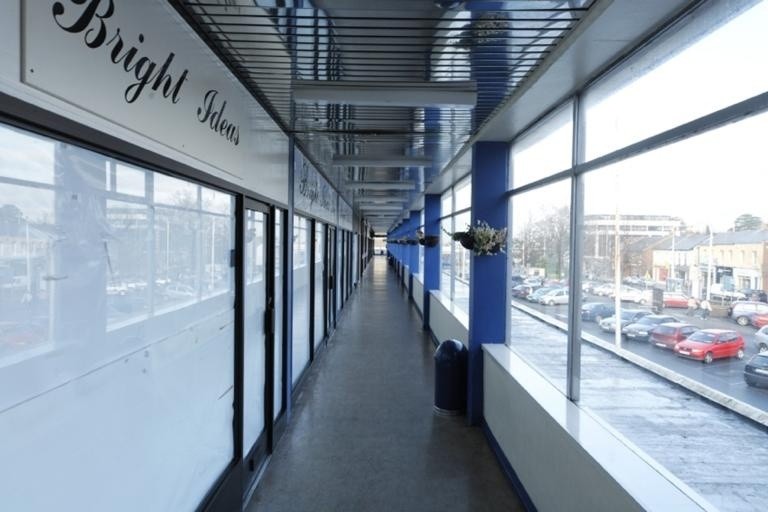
433;340;468;417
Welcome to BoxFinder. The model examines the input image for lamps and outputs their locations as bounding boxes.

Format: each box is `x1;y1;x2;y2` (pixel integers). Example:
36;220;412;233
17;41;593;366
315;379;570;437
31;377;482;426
292;78;481;233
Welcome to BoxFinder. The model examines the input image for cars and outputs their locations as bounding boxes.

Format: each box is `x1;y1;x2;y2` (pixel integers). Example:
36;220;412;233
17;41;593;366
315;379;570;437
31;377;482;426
0;275;28;291
442;255;470;281
511;272;768;390
106;258;232;301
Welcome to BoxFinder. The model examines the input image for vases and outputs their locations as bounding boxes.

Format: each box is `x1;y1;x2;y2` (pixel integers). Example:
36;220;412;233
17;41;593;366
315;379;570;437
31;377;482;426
457;231;474;250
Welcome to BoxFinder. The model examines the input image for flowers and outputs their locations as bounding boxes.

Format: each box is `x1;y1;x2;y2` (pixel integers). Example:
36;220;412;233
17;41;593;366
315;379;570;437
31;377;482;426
464;218;508;260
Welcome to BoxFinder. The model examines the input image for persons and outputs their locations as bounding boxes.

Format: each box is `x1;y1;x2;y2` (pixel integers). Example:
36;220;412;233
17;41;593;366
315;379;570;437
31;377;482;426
686;295;713;321
